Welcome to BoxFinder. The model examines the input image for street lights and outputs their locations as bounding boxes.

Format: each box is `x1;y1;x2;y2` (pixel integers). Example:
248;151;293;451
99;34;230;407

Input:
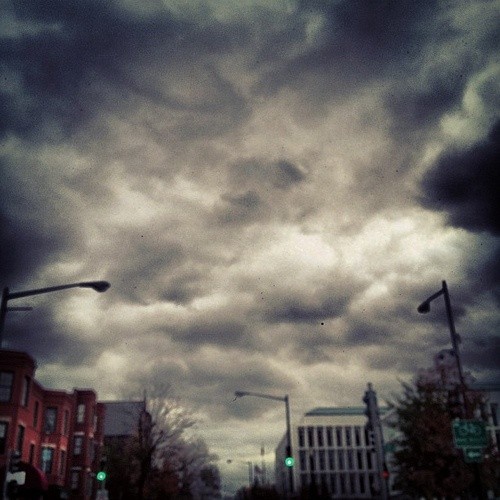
0;281;112;353
234;390;296;500
417;279;471;418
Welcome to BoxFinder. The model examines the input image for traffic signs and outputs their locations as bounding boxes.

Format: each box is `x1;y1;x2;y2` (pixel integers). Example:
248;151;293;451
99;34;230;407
450;414;492;464
